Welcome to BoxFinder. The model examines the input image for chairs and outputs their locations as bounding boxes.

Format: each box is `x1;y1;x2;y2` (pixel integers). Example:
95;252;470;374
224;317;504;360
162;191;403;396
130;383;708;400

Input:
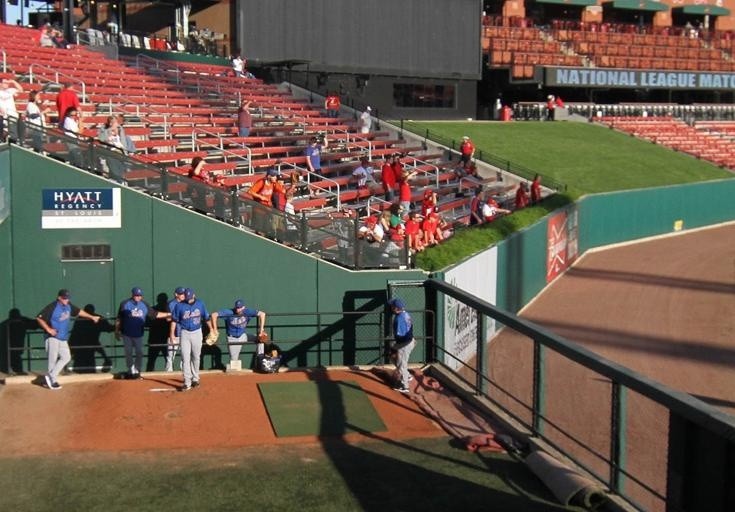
161;93;517;265
482;12;735;80
3;23;283;161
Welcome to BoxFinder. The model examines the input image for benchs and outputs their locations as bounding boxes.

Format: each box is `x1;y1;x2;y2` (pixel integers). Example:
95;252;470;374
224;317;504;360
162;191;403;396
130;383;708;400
591;115;735;170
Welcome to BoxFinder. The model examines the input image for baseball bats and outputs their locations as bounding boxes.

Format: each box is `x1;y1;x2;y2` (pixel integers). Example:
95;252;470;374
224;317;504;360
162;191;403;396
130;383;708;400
148;388;181;392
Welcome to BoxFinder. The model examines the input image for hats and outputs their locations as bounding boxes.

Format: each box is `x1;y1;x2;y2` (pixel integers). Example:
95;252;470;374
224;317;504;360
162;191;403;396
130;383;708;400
176;288;186;294
388;299;406;308
184;288;194;300
132;287;143;296
58;289;72;299
235;300;243;308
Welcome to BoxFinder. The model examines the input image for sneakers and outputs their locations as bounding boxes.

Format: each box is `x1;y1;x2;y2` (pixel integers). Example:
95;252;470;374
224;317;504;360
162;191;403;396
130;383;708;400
43;375;62;389
127;365;139;374
392;375;413;393
181;382;199;391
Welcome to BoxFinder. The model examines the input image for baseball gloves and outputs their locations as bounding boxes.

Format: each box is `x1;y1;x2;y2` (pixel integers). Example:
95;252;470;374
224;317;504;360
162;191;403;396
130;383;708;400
258;332;268;343
206;332;219;345
